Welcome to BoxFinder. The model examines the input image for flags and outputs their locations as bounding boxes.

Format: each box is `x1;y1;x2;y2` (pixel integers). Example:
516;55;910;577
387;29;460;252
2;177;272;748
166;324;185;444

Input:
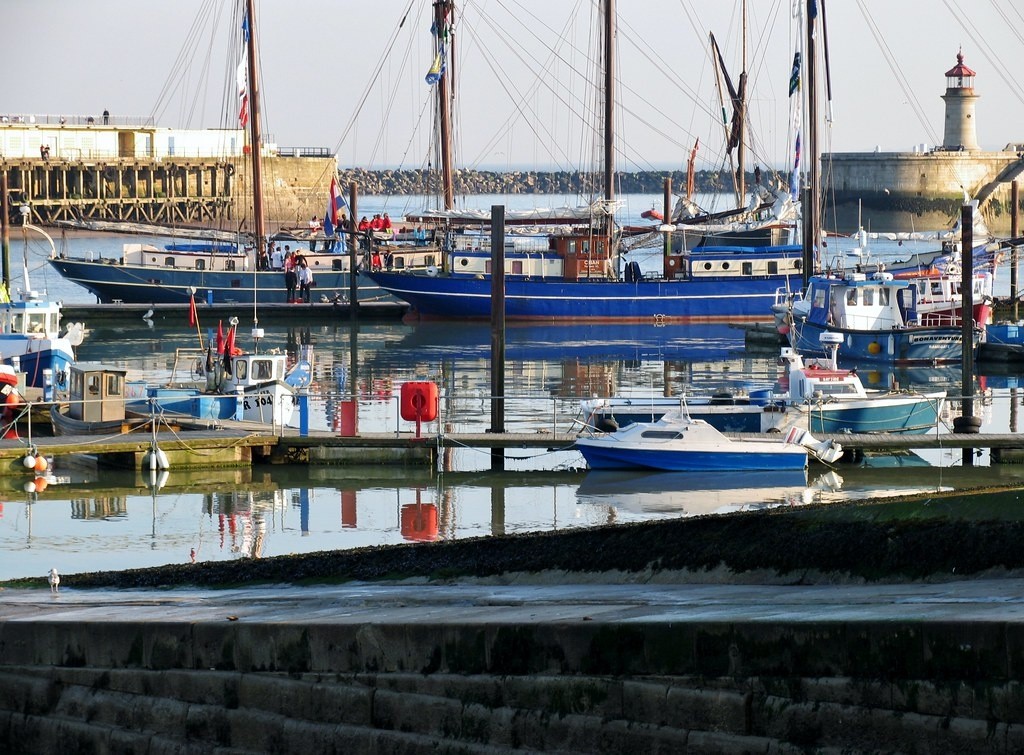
190;296;242;375
425;45;446;85
794;134;800;169
324;178;346;238
430;0;451;44
237;11;251;127
788;53;802;97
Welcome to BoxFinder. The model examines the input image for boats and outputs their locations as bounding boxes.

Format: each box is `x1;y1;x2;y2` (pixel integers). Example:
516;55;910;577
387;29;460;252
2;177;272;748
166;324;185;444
771;196;982;366
0;258;86;404
892;215;994;330
574;462;807;516
579;262;946;433
981;319;1024;361
573;375;811;472
163;269;299;423
49;361;182;437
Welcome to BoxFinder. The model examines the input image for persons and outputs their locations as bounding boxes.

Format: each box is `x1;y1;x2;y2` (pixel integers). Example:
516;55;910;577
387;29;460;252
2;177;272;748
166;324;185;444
40;144;50;161
103;109;109;125
268;242;313;303
309;213;393;271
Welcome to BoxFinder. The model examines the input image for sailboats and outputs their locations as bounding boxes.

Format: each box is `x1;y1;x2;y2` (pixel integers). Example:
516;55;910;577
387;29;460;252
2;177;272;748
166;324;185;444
845;199;866;257
264;1;1024;329
46;1;653;304
